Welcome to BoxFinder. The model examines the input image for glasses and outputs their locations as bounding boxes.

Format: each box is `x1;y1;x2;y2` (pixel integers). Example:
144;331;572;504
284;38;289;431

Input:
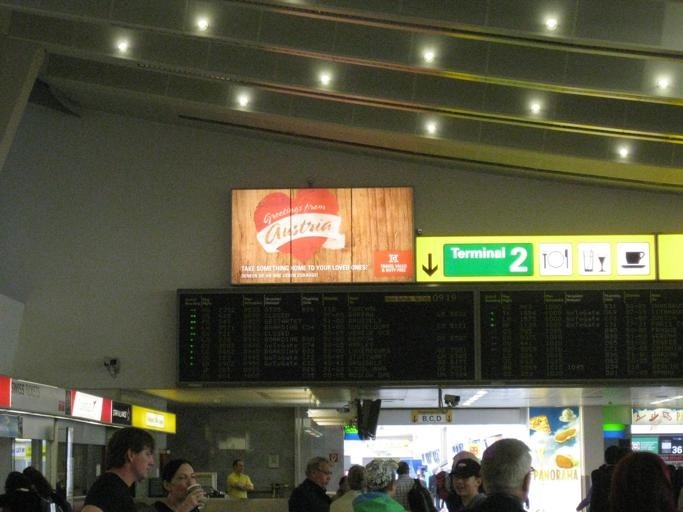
310;466;332;476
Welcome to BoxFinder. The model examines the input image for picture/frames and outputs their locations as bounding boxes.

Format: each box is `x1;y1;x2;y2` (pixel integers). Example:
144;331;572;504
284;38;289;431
267;453;280;469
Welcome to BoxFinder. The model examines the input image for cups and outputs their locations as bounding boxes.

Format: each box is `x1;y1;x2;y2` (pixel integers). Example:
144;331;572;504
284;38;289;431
185;483;208;509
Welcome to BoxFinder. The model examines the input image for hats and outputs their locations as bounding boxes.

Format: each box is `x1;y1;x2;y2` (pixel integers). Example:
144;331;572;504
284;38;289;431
365;457;400;490
448;458;482;479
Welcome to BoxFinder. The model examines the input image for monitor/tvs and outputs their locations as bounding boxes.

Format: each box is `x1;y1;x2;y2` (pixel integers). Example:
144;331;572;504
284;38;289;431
358;398;382;440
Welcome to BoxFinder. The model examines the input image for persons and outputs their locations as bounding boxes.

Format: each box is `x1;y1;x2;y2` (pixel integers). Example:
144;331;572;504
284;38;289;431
474;438;532;512
574;445;683;512
145;458;207;511
394;461;420;512
288;456;334;511
352;459;409;512
0;470;48;512
444;450;475;511
329;464;367;512
80;426;153;511
336;476;349;496
226;459;255;497
416;465;447;512
23;466;72;511
447;459;488;511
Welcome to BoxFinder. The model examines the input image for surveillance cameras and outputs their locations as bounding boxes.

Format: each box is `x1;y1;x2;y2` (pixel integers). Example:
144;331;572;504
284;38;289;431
102;358;118;367
443;394;460;406
